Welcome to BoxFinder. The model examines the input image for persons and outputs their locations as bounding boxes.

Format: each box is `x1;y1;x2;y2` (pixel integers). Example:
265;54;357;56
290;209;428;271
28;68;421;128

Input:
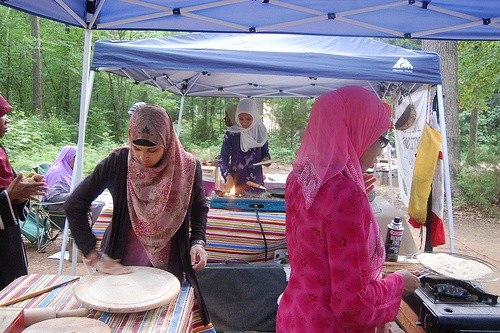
0;92;48;291
276;87;420;333
42;147;104;226
65;105;209;283
219;98;271;192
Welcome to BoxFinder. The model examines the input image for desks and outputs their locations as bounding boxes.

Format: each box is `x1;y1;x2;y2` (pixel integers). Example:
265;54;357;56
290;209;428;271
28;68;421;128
0;274;217;333
202;173;225;196
264;174;288;194
91;196;418;265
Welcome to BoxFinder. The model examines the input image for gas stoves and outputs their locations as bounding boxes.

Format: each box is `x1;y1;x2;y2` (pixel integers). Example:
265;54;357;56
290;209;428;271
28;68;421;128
209;189;286;212
401;276;500;333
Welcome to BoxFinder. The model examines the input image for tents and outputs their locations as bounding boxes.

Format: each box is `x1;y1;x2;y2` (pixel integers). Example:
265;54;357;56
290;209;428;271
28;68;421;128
58;31;457;257
0;0;500;275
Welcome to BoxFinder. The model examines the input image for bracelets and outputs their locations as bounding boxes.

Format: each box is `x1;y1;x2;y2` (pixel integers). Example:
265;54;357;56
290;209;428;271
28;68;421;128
189;240;205;254
85;253;107;269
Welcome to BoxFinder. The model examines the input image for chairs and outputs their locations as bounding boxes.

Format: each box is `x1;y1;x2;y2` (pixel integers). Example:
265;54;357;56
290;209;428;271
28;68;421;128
194;263;286;333
31;163;92;253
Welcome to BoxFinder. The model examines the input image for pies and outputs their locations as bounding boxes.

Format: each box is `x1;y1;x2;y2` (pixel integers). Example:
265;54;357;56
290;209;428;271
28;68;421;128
90;272;171;303
253;160;276;165
416;252;494;280
21;316;111;333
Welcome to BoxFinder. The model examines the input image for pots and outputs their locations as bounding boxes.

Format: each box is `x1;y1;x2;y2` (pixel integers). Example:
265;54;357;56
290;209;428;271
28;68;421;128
245;180;286;198
